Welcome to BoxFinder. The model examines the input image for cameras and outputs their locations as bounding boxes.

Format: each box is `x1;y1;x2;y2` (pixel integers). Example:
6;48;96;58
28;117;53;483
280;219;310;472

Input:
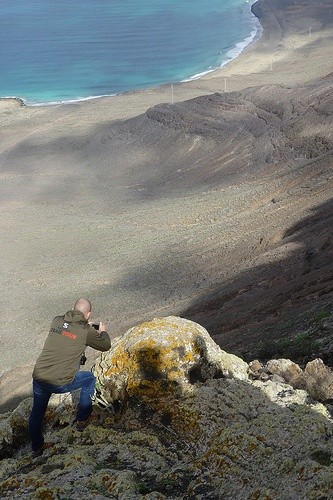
91;324;100;331
80;356;87;365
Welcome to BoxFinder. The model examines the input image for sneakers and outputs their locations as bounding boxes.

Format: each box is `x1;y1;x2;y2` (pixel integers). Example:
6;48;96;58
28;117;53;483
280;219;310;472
32;442;54;458
76;416;97;431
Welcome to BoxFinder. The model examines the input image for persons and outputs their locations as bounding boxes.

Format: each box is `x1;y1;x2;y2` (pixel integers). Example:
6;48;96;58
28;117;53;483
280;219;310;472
29;298;111;458
